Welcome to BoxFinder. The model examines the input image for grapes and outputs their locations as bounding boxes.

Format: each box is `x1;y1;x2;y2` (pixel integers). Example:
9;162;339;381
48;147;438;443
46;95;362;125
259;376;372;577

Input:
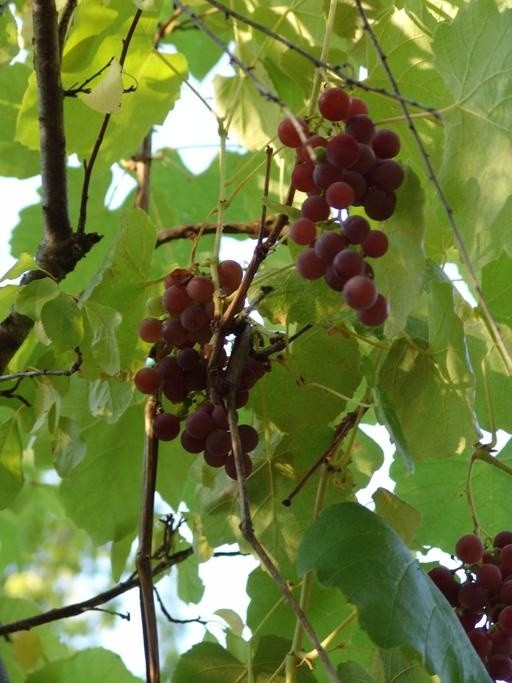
278;88;402;325
134;260;260;480
427;533;512;683
